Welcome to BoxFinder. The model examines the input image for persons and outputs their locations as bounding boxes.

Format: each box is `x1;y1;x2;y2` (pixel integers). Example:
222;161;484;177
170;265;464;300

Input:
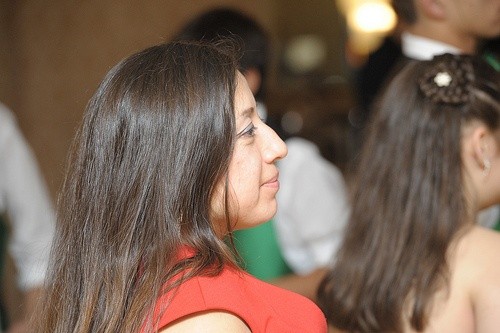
0;0;500;333
